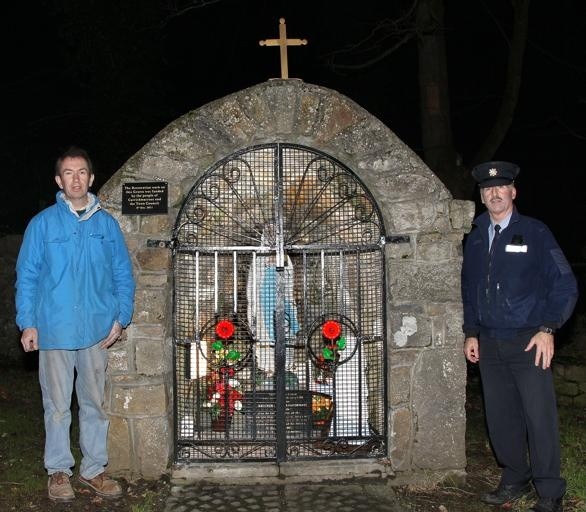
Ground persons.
[244,224,300,377]
[14,145,135,501]
[460,160,579,510]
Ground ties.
[490,224,502,251]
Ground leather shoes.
[77,469,124,497]
[47,471,77,504]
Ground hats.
[469,160,523,189]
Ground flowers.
[311,318,346,389]
[206,318,245,404]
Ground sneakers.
[480,476,533,506]
[525,495,563,512]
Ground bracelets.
[538,326,556,335]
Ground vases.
[207,400,235,432]
